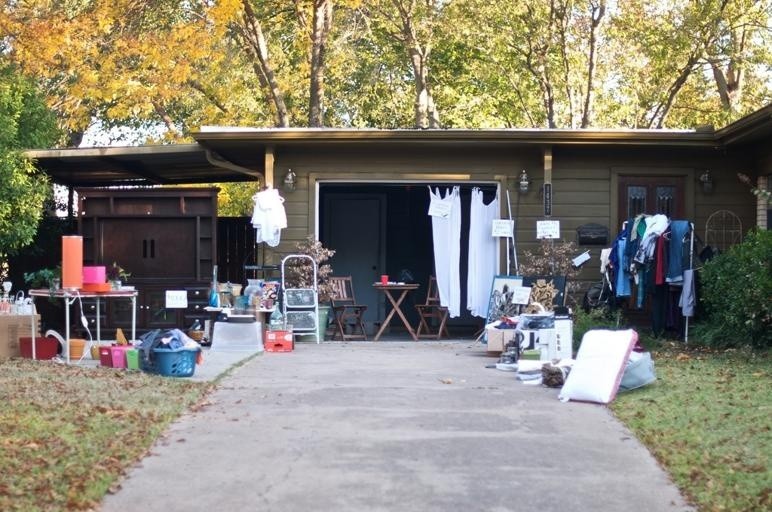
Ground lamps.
[697,165,716,196]
[282,167,300,196]
[60,234,83,291]
[513,168,534,197]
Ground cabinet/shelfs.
[72,186,222,342]
[215,213,258,289]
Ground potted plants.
[283,234,339,344]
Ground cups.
[380,276,388,285]
[49,277,59,292]
[115,280,122,291]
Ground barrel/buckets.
[69,338,86,361]
[125,349,139,369]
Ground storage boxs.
[123,347,148,371]
[1,313,45,363]
[98,345,134,369]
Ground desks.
[200,305,280,340]
[24,286,139,366]
[370,281,421,342]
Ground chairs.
[412,274,451,342]
[279,251,322,346]
[325,272,372,341]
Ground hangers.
[252,181,286,204]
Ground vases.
[108,279,122,289]
[244,277,263,297]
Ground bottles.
[0,292,33,315]
[187,319,203,343]
[270,302,283,331]
[501,347,517,364]
[210,265,280,317]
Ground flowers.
[102,261,133,283]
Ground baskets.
[135,341,201,378]
